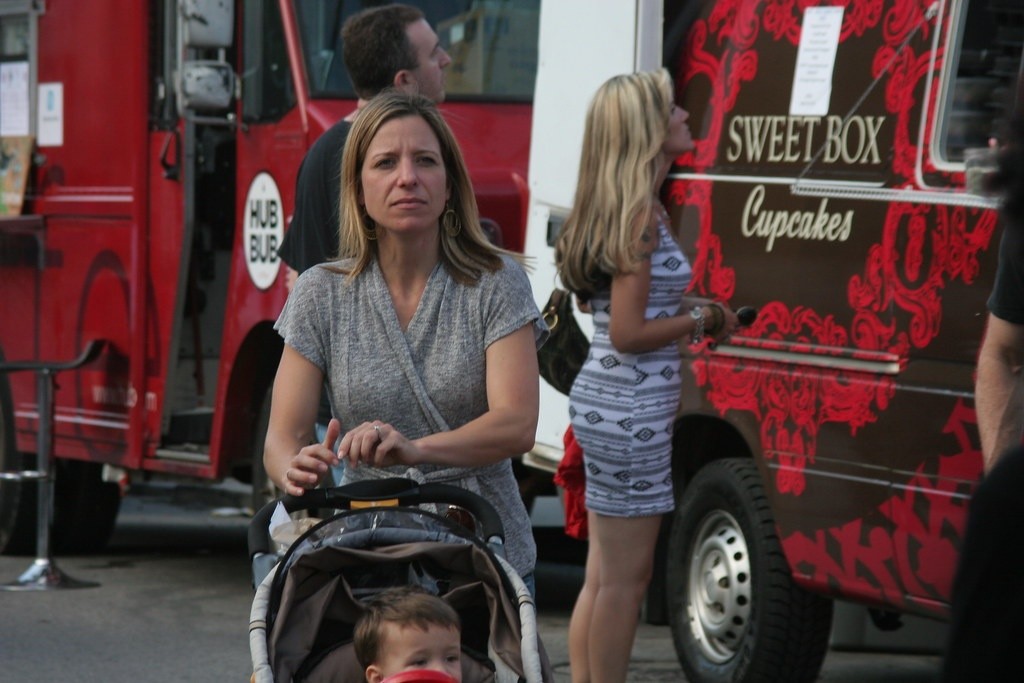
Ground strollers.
[245,477,555,683]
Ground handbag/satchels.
[537,265,592,396]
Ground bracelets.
[689,305,705,345]
[706,304,725,335]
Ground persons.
[266,2,453,298]
[352,587,466,679]
[943,2,1024,683]
[554,66,739,679]
[265,86,546,606]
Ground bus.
[510,0,1024,683]
[0,0,541,559]
